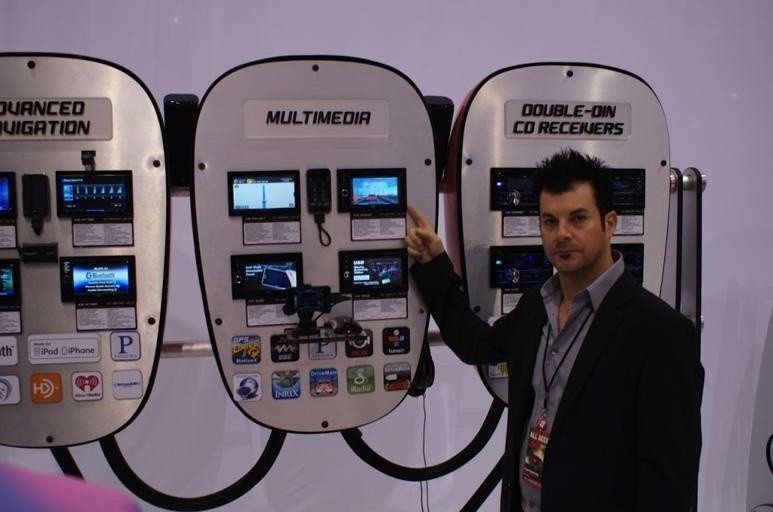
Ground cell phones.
[285,285,331,312]
[20,174,50,218]
[307,167,332,213]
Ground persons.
[406,146,705,511]
